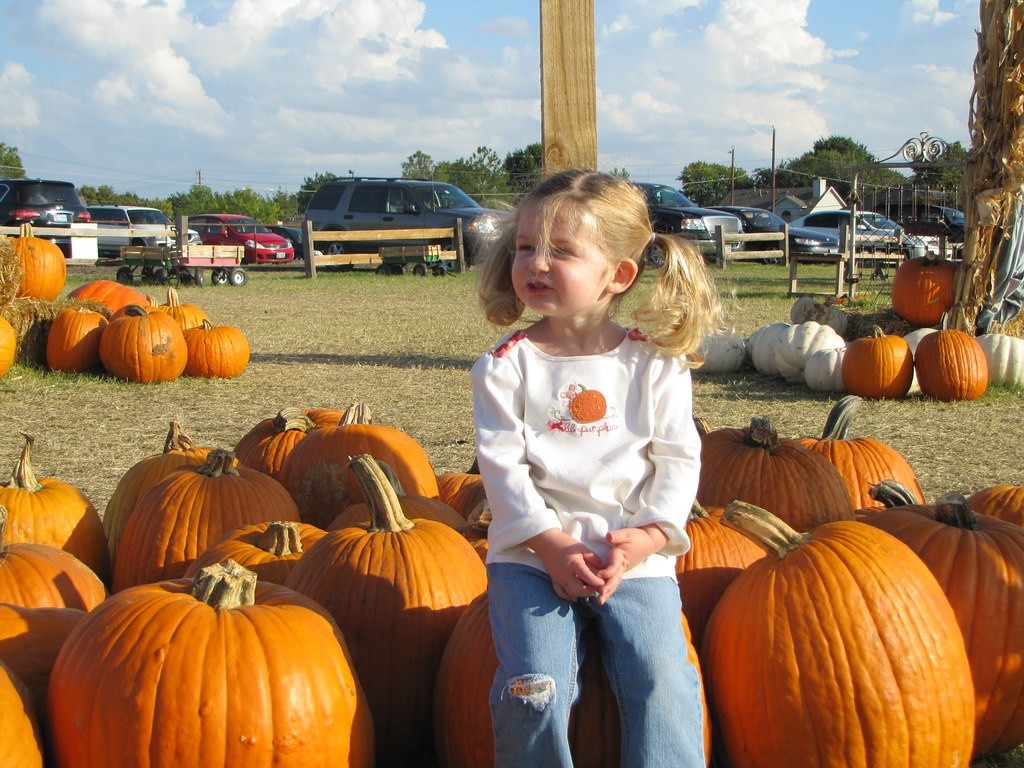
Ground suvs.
[86,204,203,257]
[300,176,514,272]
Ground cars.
[0,178,91,268]
[636,183,744,270]
[878,200,966,259]
[790,211,927,267]
[187,215,294,265]
[708,206,838,265]
[267,226,304,260]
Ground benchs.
[787,223,904,300]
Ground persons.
[470,156,743,768]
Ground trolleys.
[117,227,250,286]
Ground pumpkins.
[6,222,67,301]
[46,280,250,383]
[677,395,1024,768]
[688,251,1024,402]
[0,403,621,768]
[0,316,16,378]
